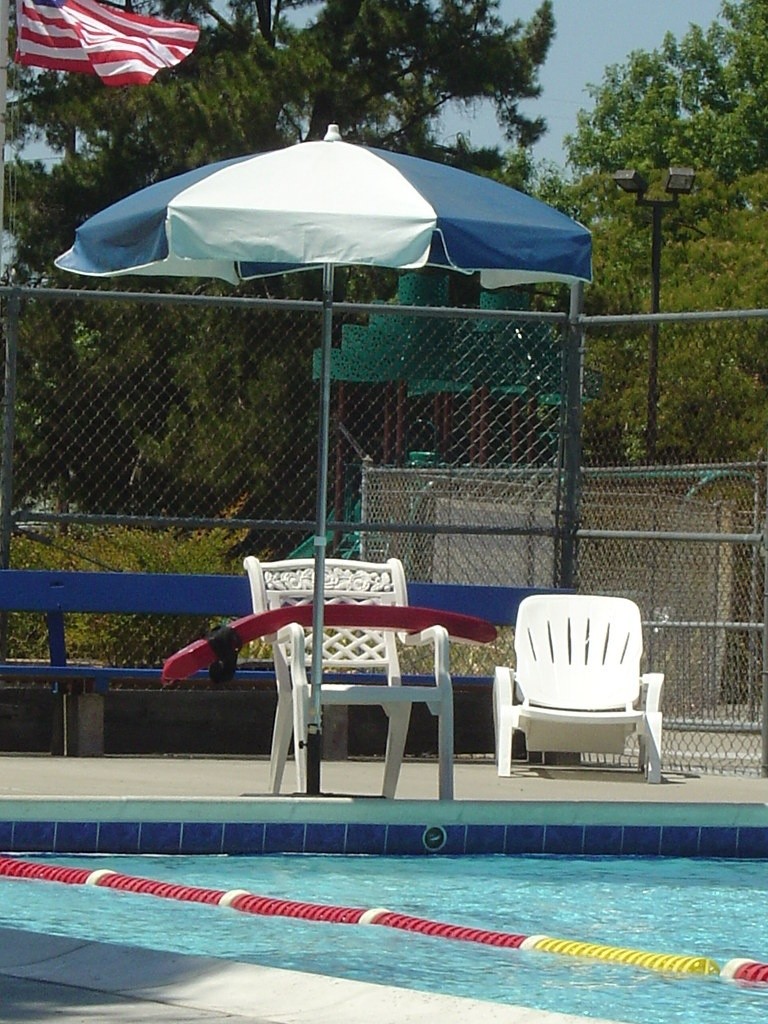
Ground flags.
[13,0,199,86]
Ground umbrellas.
[55,125,592,794]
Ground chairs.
[492,594,662,785]
[244,557,455,799]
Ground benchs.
[0,568,583,766]
